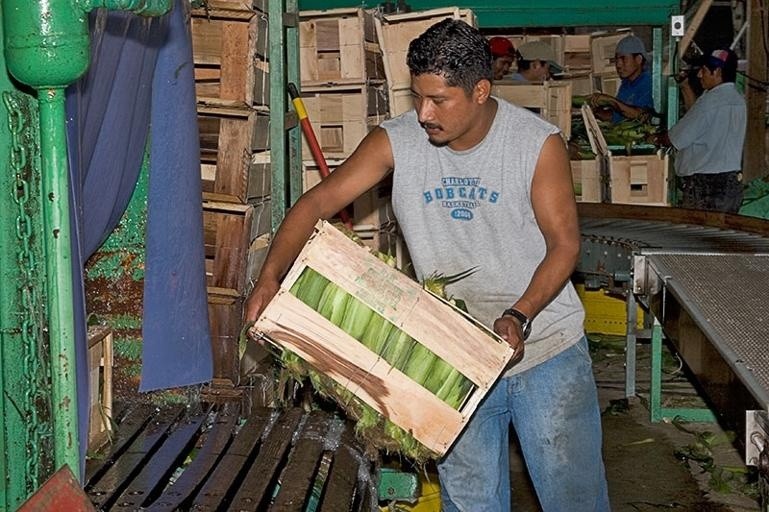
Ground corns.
[277,221,476,464]
[568,95,660,197]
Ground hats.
[704,46,737,68]
[489,37,515,57]
[517,41,565,73]
[616,36,650,63]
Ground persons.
[512,40,565,114]
[654,47,748,214]
[585,34,653,125]
[489,36,515,80]
[244,18,611,512]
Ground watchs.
[502,308,532,338]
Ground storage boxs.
[254,221,515,459]
[88,326,112,460]
[83,0,687,407]
